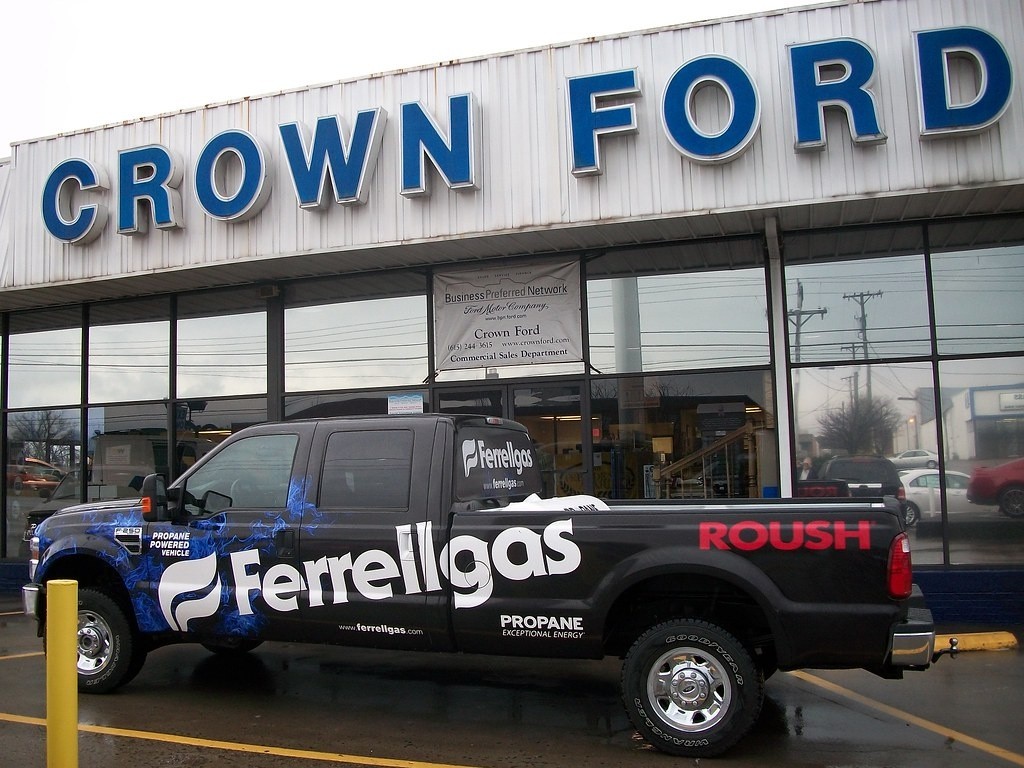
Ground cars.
[966,457,1024,518]
[5,455,72,500]
[887,450,940,469]
[897,470,1012,527]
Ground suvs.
[23,465,164,555]
[807,453,907,523]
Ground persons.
[796,456,816,480]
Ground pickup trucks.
[22,411,958,759]
